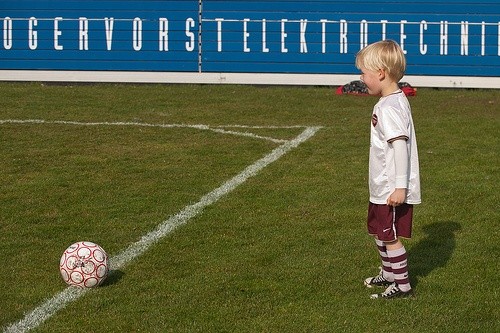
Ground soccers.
[58,242,110,290]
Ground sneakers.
[370,282,414,301]
[363,274,395,288]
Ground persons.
[356,41,422,300]
